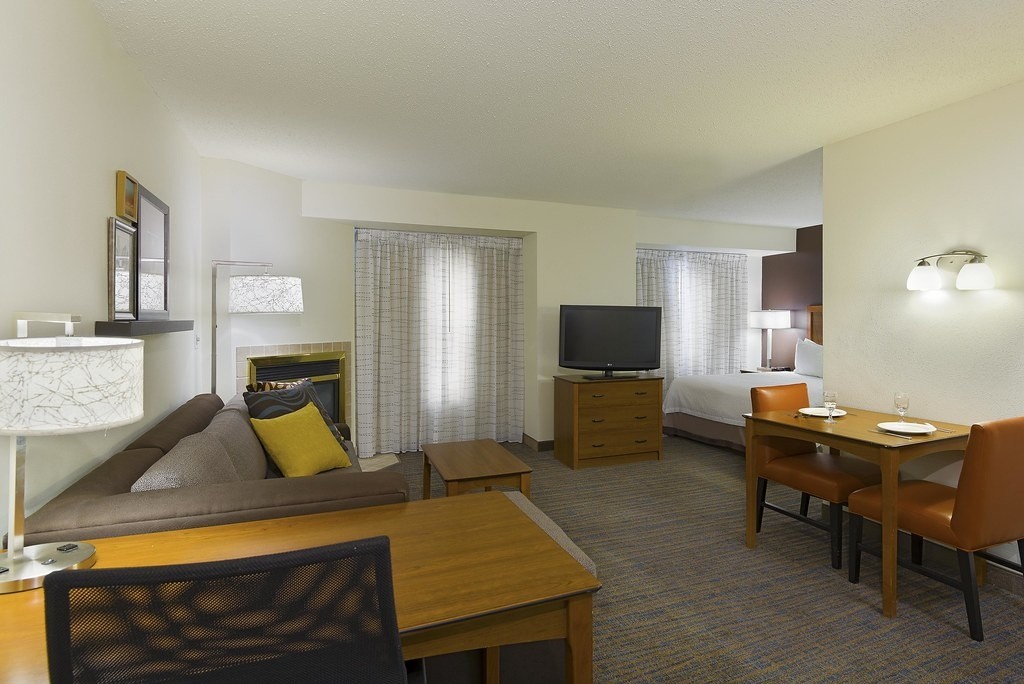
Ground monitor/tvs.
[559,305,662,380]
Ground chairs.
[42,537,403,683]
[750,384,902,568]
[847,417,1024,642]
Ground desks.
[740,404,972,615]
[0,491,604,684]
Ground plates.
[877,422,938,433]
[798,407,847,417]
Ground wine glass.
[822,391,838,423]
[893,392,910,428]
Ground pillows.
[250,402,353,478]
[246,377,345,442]
[242,379,349,480]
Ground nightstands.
[740,366,794,374]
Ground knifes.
[868,430,912,440]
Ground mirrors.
[133,184,170,319]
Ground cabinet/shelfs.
[552,372,663,471]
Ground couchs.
[3,394,409,550]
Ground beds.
[664,305,823,452]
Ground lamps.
[0,312,143,594]
[749,309,790,370]
[211,260,302,394]
[905,249,994,293]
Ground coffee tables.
[419,439,532,499]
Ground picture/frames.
[106,216,137,320]
[114,171,138,220]
